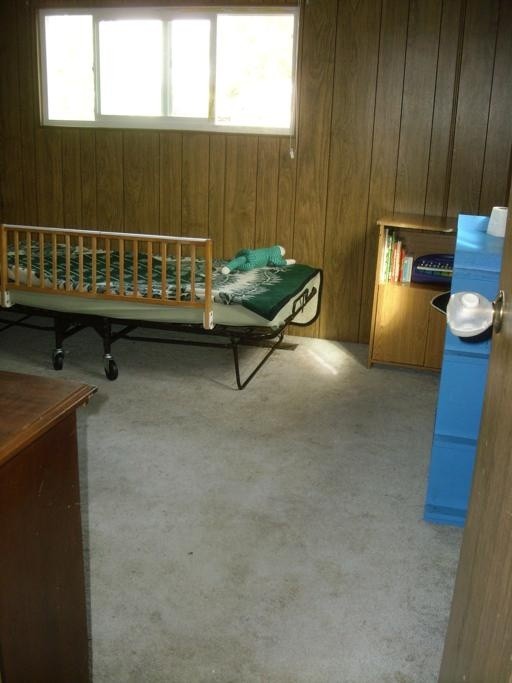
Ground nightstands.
[366,214,459,375]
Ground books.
[382,228,413,284]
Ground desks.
[0,370,99,683]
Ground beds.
[0,221,325,391]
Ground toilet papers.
[486,206,508,239]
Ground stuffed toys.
[222,244,299,275]
